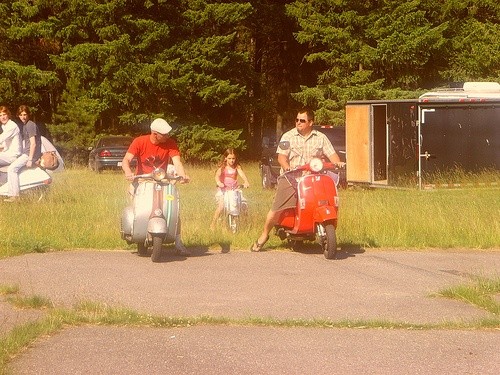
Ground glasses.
[295,119,305,123]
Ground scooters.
[0,145,60,196]
[121,156,187,264]
[273,143,347,258]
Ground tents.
[22,136,66,172]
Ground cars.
[85,135,140,172]
[258,125,347,191]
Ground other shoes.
[4,196,20,203]
[177,245,191,256]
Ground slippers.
[250,235,269,252]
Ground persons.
[4,105,41,202]
[208,147,249,232]
[0,106,23,167]
[122,118,191,256]
[249,108,344,252]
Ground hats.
[150,118,172,134]
